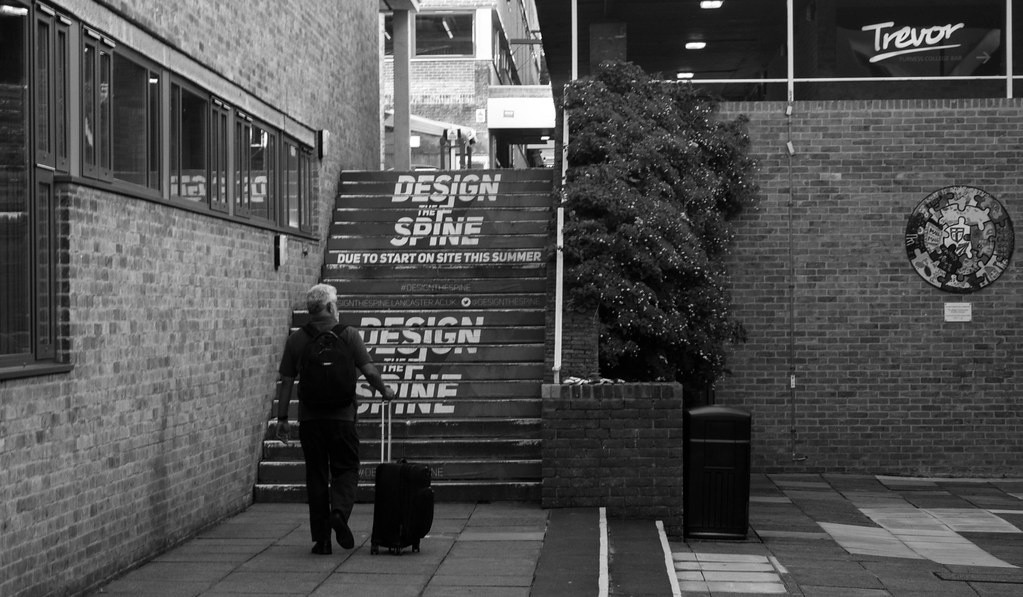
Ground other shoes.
[329,512,355,550]
[311,538,333,556]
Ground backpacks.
[297,319,359,421]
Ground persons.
[276,284,394,554]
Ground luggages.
[370,400,435,555]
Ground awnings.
[487,97,556,129]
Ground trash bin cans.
[683,406,752,541]
